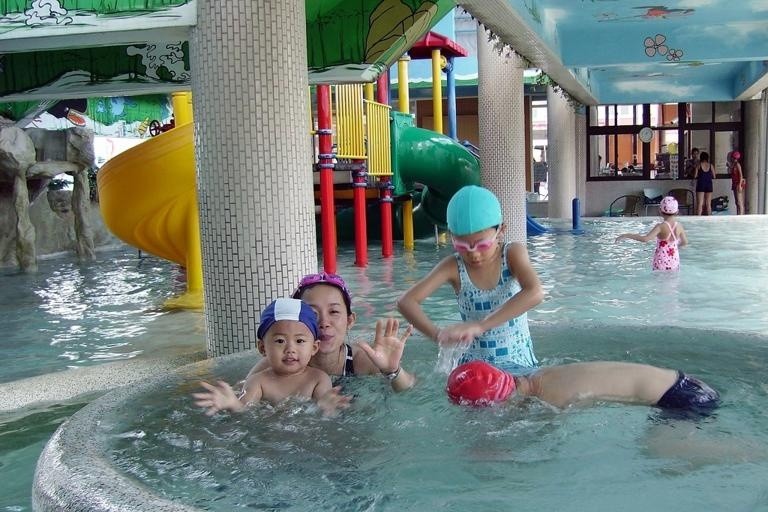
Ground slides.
[314,127,479,242]
[97,120,202,265]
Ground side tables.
[642,203,661,216]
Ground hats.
[731,150,741,159]
[288,272,353,308]
[659,196,679,214]
[445,361,519,410]
[255,296,321,340]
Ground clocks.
[639,127,654,143]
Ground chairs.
[609,195,641,217]
[668,189,695,215]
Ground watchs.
[386,366,402,381]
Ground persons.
[243,271,415,394]
[726,151,745,215]
[694,152,716,215]
[394,186,544,374]
[446,360,766,475]
[614,195,689,273]
[685,148,700,179]
[620,164,636,174]
[191,297,352,420]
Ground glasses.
[450,224,503,254]
[299,271,347,289]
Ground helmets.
[445,184,503,236]
